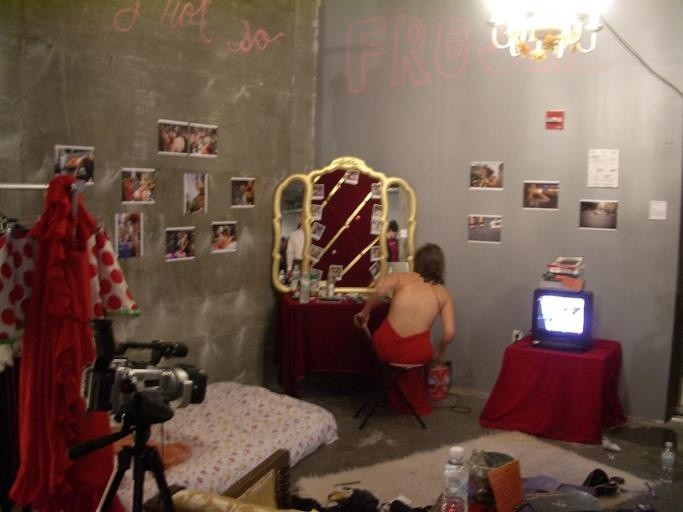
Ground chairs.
[352,325,428,430]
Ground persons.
[385,220,401,263]
[59,125,255,261]
[479,167,496,188]
[525,184,551,207]
[352,242,456,417]
[470,216,484,228]
[285,223,306,281]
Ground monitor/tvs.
[532,287,594,352]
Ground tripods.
[94,444,175,512]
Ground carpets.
[296,429,656,512]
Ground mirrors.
[270,156,417,296]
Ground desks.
[279,294,391,398]
[502,334,620,443]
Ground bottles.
[291,264,300,288]
[442,447,469,512]
[660,442,675,485]
[328,273,336,297]
[299,271,319,303]
